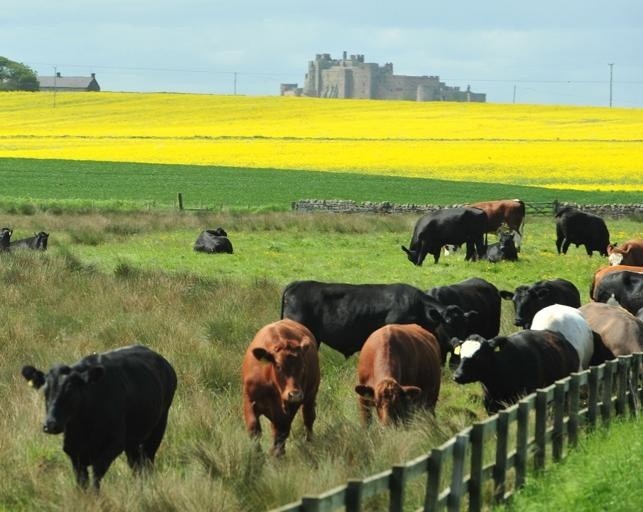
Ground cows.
[531,304,595,393]
[449,329,581,417]
[0,228,13,254]
[442,198,526,257]
[552,205,618,258]
[576,303,642,382]
[241,317,320,457]
[401,207,489,267]
[355,323,440,432]
[425,278,503,340]
[9,227,49,252]
[192,227,234,254]
[589,265,643,315]
[22,345,177,493]
[499,277,582,330]
[280,280,464,370]
[472,231,520,262]
[607,240,642,264]
